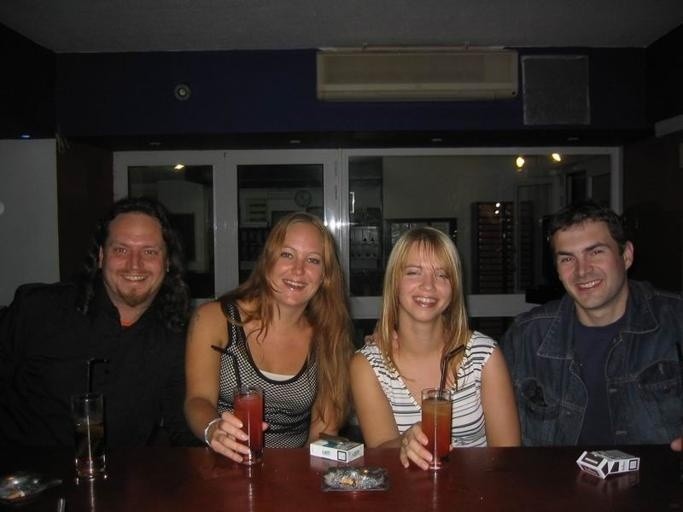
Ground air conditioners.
[315,48,517,100]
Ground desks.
[1,444,649,512]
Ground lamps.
[514,154,575,171]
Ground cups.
[421,387,453,471]
[232,385,264,467]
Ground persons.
[502,199,681,452]
[0,196,206,468]
[346,227,522,470]
[185,212,355,464]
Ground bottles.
[69,391,108,485]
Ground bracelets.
[204,418,220,444]
